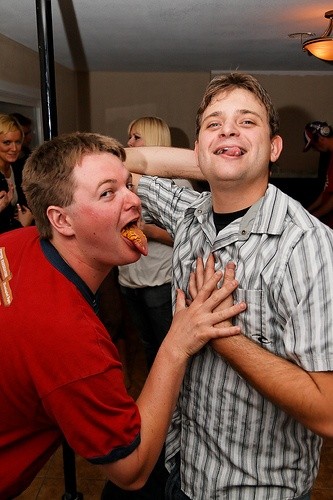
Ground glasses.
[308,143,315,148]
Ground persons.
[108,117,195,367]
[296,121,333,220]
[0,109,39,227]
[117,71,333,500]
[0,133,250,500]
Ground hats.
[302,121,326,153]
